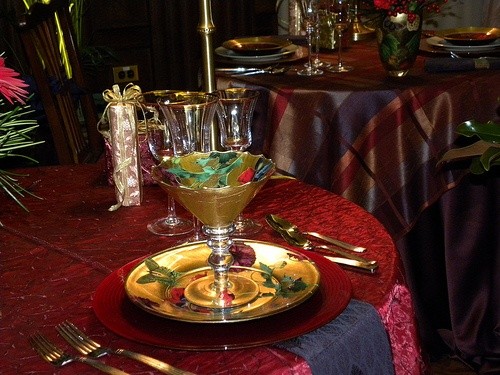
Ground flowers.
[356,0,449,29]
[0,50,43,214]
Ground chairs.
[17,0,107,165]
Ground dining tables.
[0,164,419,375]
[215,29,500,371]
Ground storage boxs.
[97,84,159,211]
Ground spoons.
[266,214,366,253]
[217,65,290,77]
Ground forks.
[27,332,131,375]
[280,230,377,273]
[54,320,196,375]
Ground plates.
[214,44,298,61]
[124,237,322,323]
[221,35,292,55]
[438,25,500,43]
[427,37,500,51]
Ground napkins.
[427,57,500,73]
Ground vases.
[376,10,424,77]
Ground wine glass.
[136,87,263,237]
[150,148,277,309]
[296,3,354,76]
